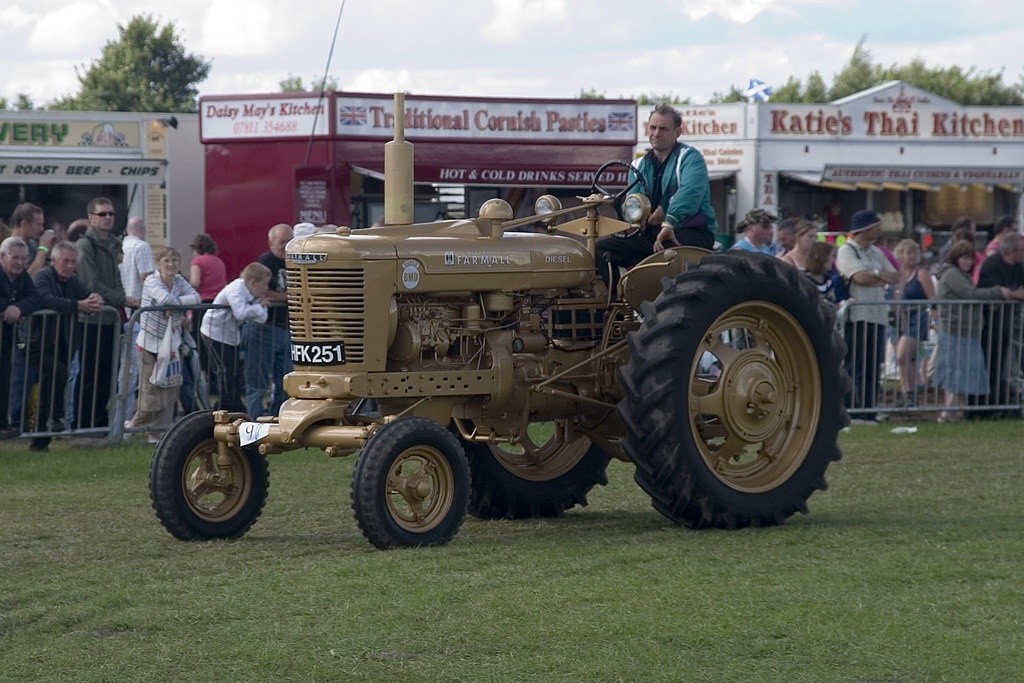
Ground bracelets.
[873,269,880,278]
[36,246,49,254]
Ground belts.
[266,320,289,329]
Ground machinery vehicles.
[146,91,854,551]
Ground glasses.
[5,253,30,262]
[90,211,115,217]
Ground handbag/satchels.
[826,243,860,301]
[149,316,185,389]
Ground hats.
[745,207,782,224]
[850,208,884,234]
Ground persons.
[595,106,719,304]
[0,194,1024,448]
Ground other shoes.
[0,424,22,440]
[123,420,134,441]
[896,390,917,407]
[147,433,159,443]
[875,412,890,423]
[27,441,49,453]
[47,418,66,433]
[938,410,966,425]
